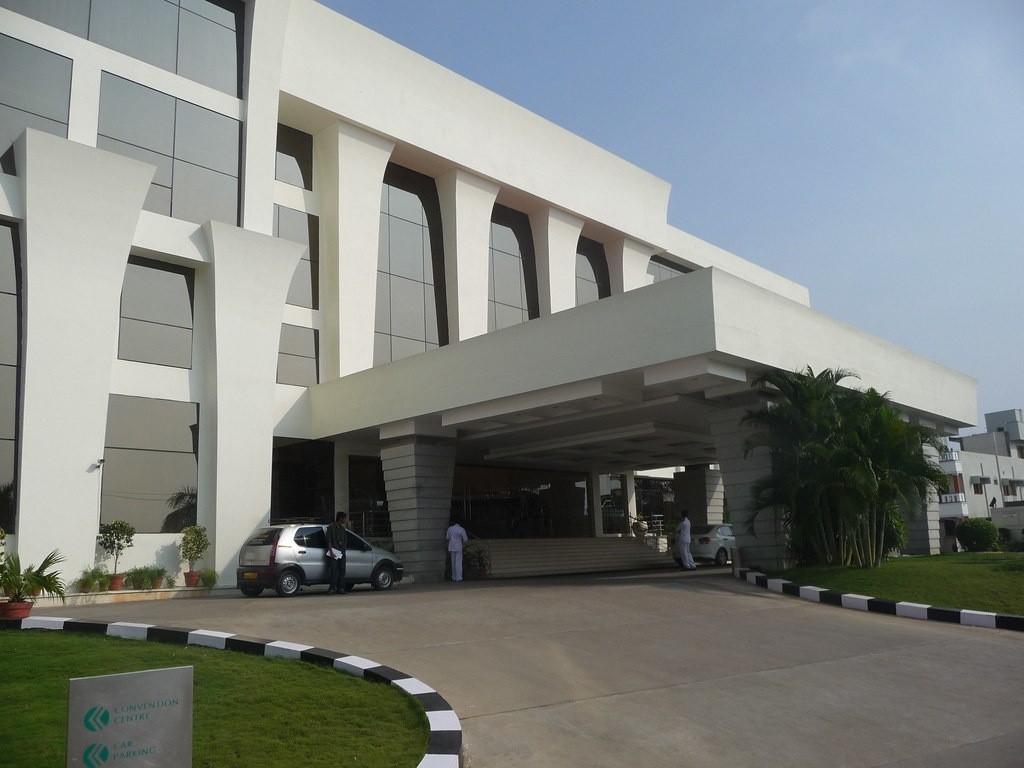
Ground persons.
[446,517,468,582]
[512,510,521,538]
[327,512,347,595]
[675,511,696,570]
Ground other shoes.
[337,589,346,594]
[681,567,696,571]
[327,590,336,595]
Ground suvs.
[236,517,404,598]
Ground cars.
[670,523,735,568]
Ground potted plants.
[461,543,490,581]
[632,514,648,537]
[179,525,211,588]
[0,546,67,620]
[76,521,166,591]
